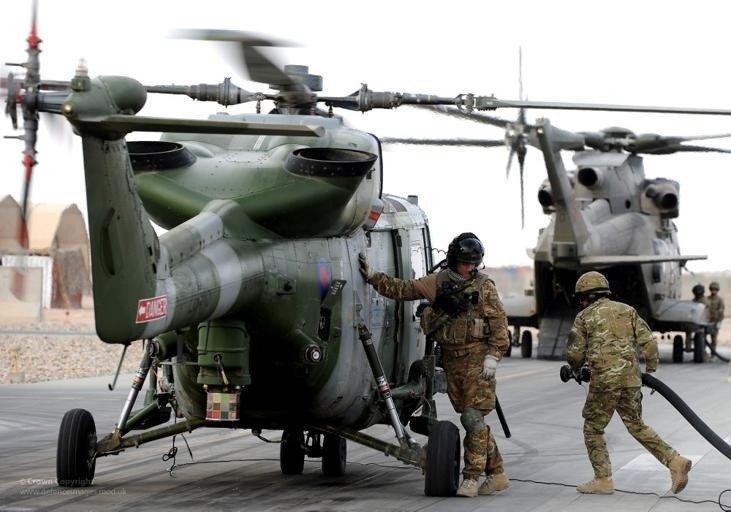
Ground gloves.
[358,252,377,280]
[481,355,498,379]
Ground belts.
[442,342,478,358]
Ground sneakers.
[577,476,616,495]
[457,477,479,497]
[478,472,510,494]
[668,455,693,493]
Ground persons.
[567,271,691,495]
[359,233,510,497]
[693,281,725,363]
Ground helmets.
[692,284,705,296]
[709,282,721,291]
[572,270,611,298]
[447,232,486,267]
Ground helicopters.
[377,44,731,365]
[1,2,463,495]
